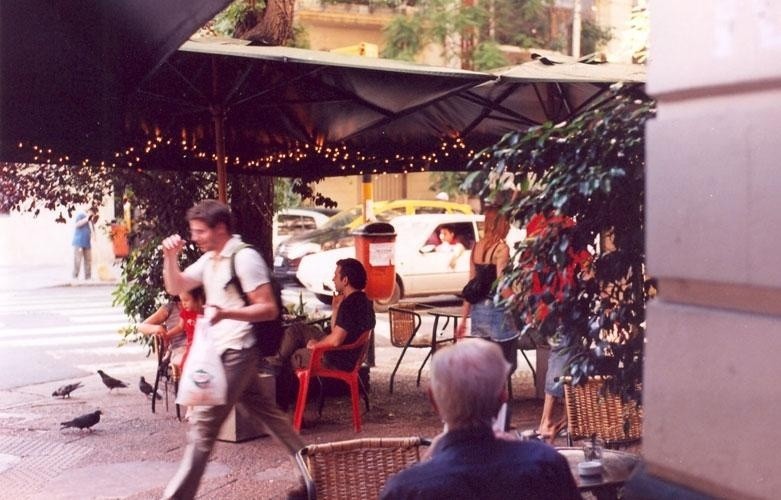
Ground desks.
[427,307,537,400]
[553,446,638,500]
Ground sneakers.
[254,355,283,376]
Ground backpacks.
[223,245,285,358]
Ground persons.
[157,200,318,500]
[419,224,468,267]
[521,204,609,442]
[161,287,206,373]
[458,207,536,431]
[68,203,98,286]
[141,294,188,363]
[378,333,586,500]
[258,255,377,430]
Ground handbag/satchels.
[463,276,493,304]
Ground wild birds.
[97,371,127,395]
[139,377,161,401]
[60,411,103,436]
[52,381,84,399]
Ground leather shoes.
[286,483,308,497]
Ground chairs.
[553,376,644,454]
[296,437,433,500]
[149,325,183,422]
[279,301,455,435]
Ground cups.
[583,439,603,464]
